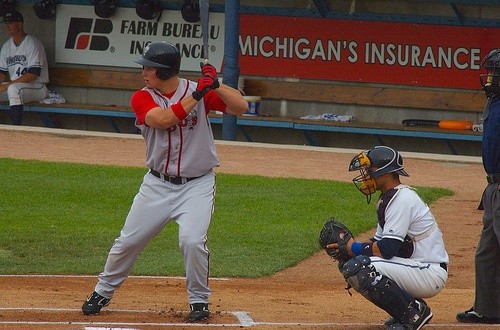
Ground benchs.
[0,68,486,155]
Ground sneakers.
[456,306,500,324]
[81,291,111,314]
[385,298,433,330]
[186,304,209,322]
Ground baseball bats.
[199,0,209,64]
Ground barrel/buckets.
[243,95,260,117]
[216,77,246,115]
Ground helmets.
[132,41,181,81]
[480,49,500,100]
[366,145,410,177]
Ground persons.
[327,145,449,330]
[455,49,500,326]
[82,41,248,324]
[0,10,50,126]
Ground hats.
[0,11,24,23]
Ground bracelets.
[361,243,373,256]
[351,241,362,256]
[170,99,187,121]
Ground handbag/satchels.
[477,183,489,210]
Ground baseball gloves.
[318,219,355,261]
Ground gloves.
[191,74,214,101]
[200,62,220,89]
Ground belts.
[486,175,500,185]
[439,263,448,271]
[150,169,203,184]
